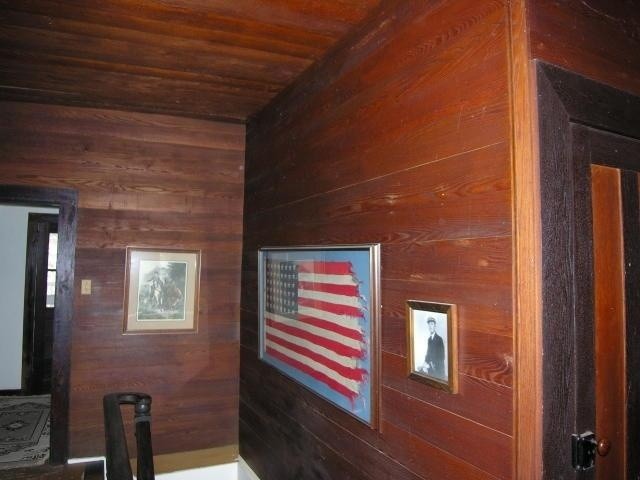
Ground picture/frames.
[257,244,383,432]
[121,245,203,337]
[403,299,458,395]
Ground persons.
[416,316,446,383]
[151,271,164,310]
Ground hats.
[427,317,437,323]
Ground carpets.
[0,393,51,472]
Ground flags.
[264,255,373,416]
